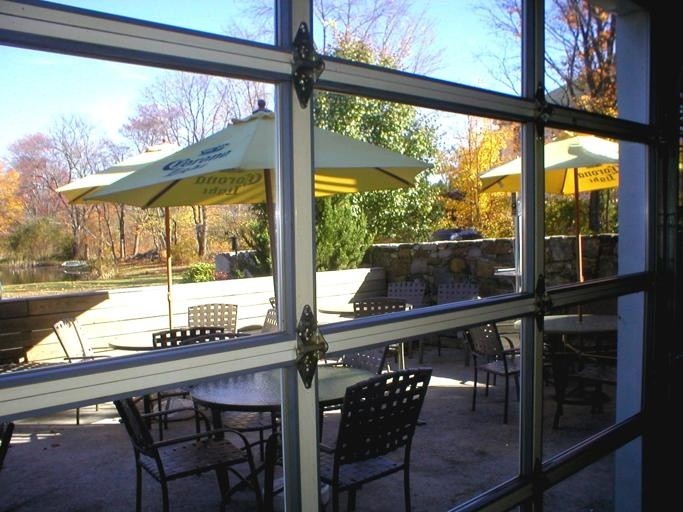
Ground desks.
[319,304,411,365]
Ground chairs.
[353,278,618,433]
[50,304,434,511]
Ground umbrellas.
[480,134,619,321]
[55,139,184,328]
[83,100,434,321]
